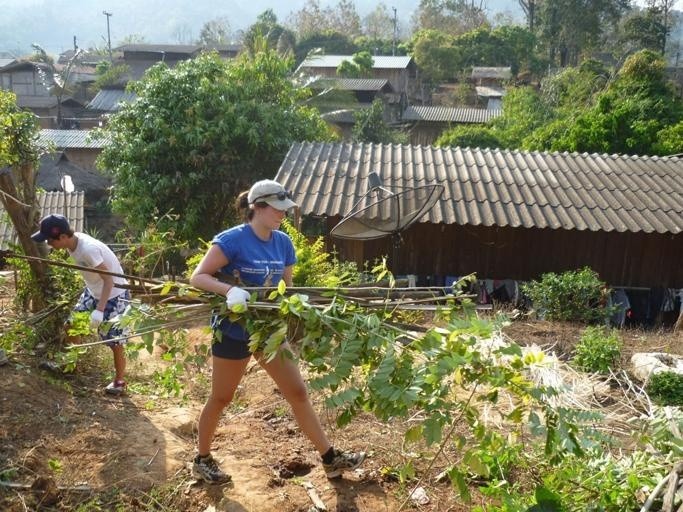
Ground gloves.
[225,286,250,313]
[87,308,103,330]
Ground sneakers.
[322,447,365,479]
[39,357,67,372]
[102,379,126,394]
[190,454,233,485]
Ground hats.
[29,213,70,243]
[246,178,298,212]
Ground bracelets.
[227,285,233,292]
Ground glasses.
[251,189,293,203]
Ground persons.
[30,212,133,397]
[188,179,371,487]
